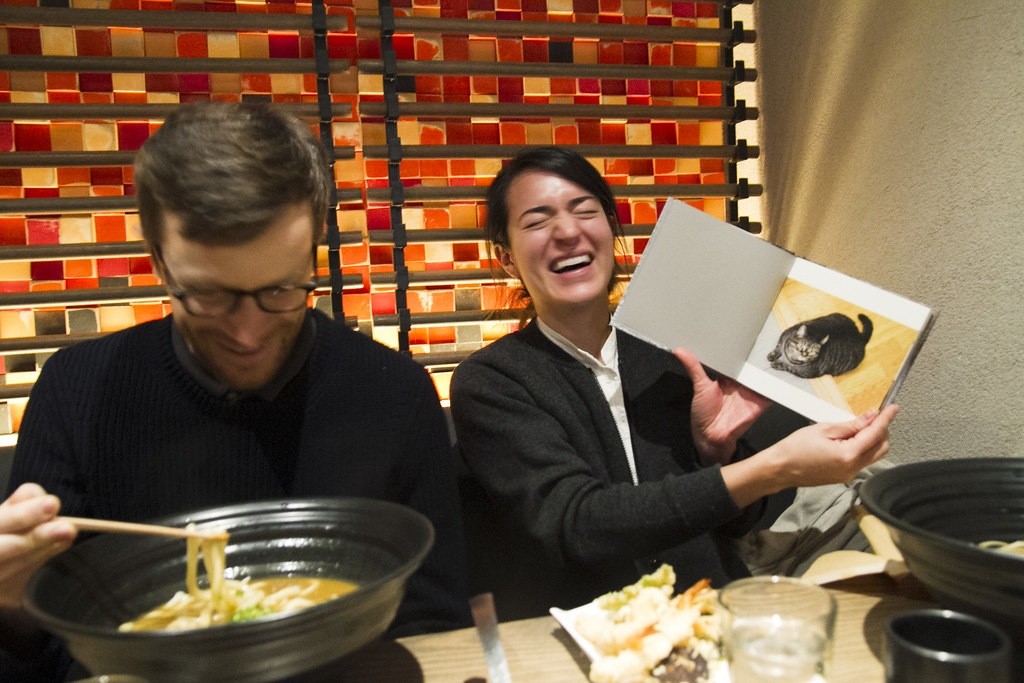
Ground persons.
[447,143,899,620]
[0,99,466,683]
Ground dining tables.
[397,570,1024,683]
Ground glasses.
[151,243,319,317]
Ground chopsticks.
[49,515,230,541]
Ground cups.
[884,608,1012,683]
[717,576,836,683]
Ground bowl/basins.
[860,458,1024,634]
[22,496,436,683]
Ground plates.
[549,584,830,683]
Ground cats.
[767,313,873,379]
